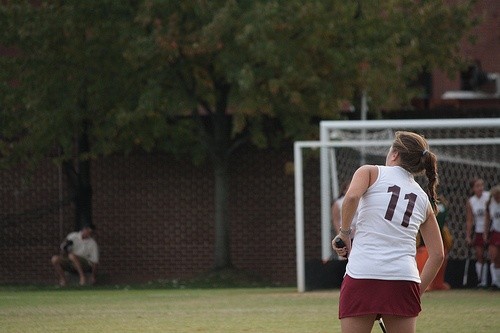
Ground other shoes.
[78,282,84,289]
[492,285,499,290]
[55,284,63,289]
[476,284,486,290]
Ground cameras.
[64,240,73,249]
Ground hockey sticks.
[462,224,475,286]
[334,238,391,333]
